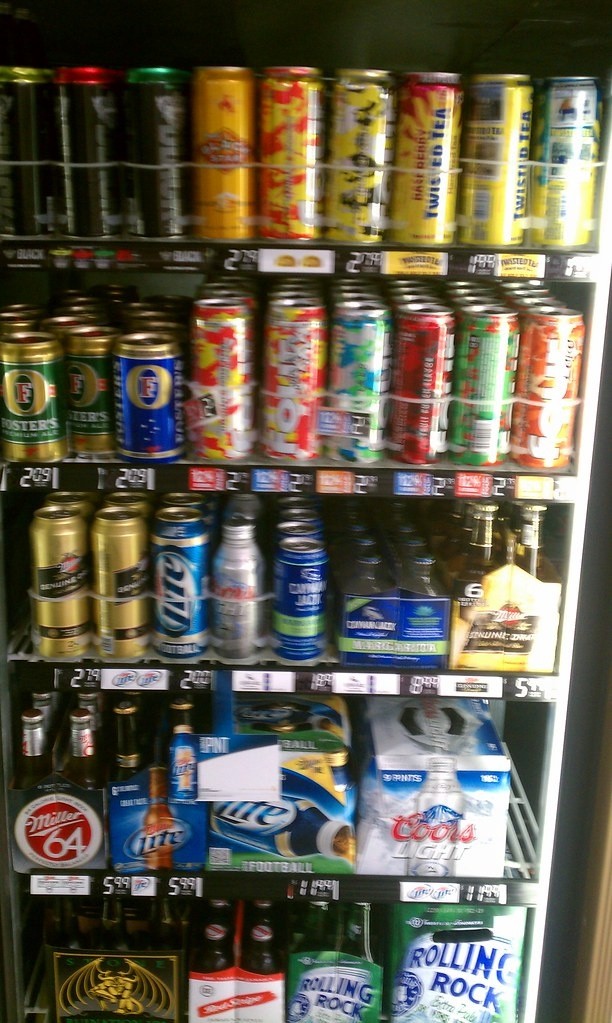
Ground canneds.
[1,68,602,663]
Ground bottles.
[7,681,205,884]
[22,890,384,1023]
[332,492,561,672]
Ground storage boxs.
[449,551,563,674]
[105,762,207,874]
[326,588,452,674]
[187,898,285,1023]
[206,693,355,875]
[283,902,382,1022]
[353,693,513,880]
[5,732,111,873]
[387,901,529,1023]
[39,942,188,1023]
